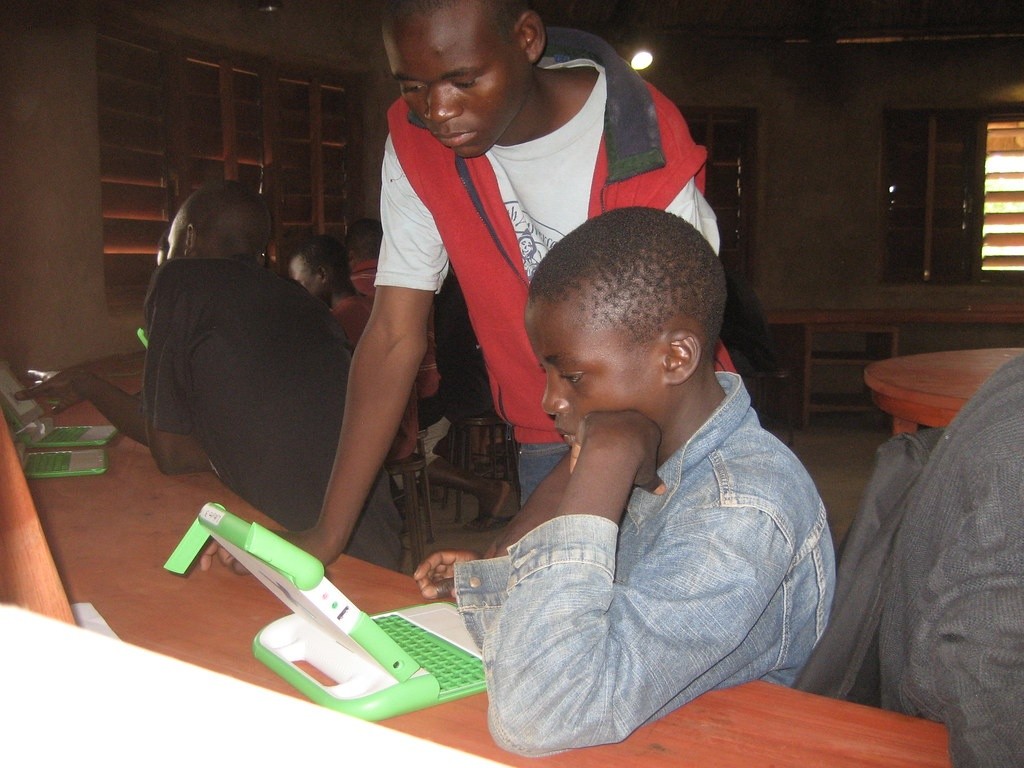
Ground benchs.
[759,306,1024,429]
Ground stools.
[739,366,793,446]
[387,452,433,573]
[441,412,520,525]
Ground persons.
[137,178,513,575]
[198,0,796,577]
[411,207,840,758]
[712,267,790,444]
[879,354,1024,768]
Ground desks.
[0,397,951,767]
[863,348,1024,434]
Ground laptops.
[163,501,493,723]
[0,360,119,479]
